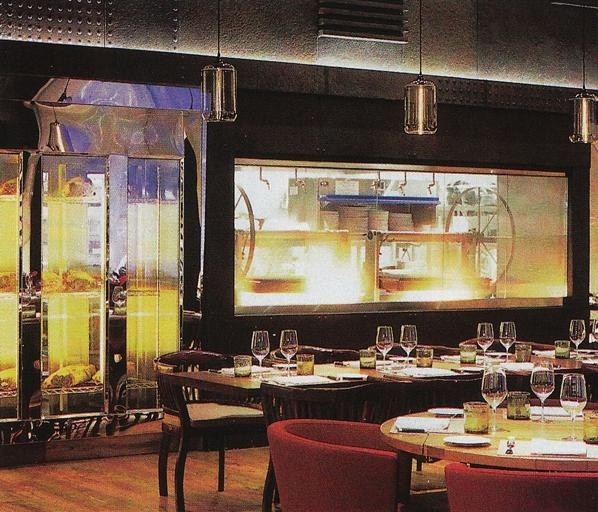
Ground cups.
[295,354,315,375]
[233,355,252,379]
[508,390,531,422]
[554,340,571,359]
[582,413,598,444]
[360,348,378,369]
[464,401,490,432]
[514,344,532,361]
[459,344,477,366]
[415,346,435,367]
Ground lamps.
[566,1,598,146]
[201,1,238,125]
[402,0,438,138]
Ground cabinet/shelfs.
[235,229,498,304]
[0,148,186,448]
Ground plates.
[319,203,415,234]
[428,408,465,415]
[444,435,490,446]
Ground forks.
[504,438,516,455]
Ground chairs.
[153,339,597,511]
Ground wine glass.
[477,322,496,366]
[279,329,299,379]
[528,367,555,425]
[399,324,419,368]
[499,322,516,364]
[482,367,508,424]
[251,331,270,379]
[569,319,586,360]
[376,326,395,371]
[560,373,588,442]
